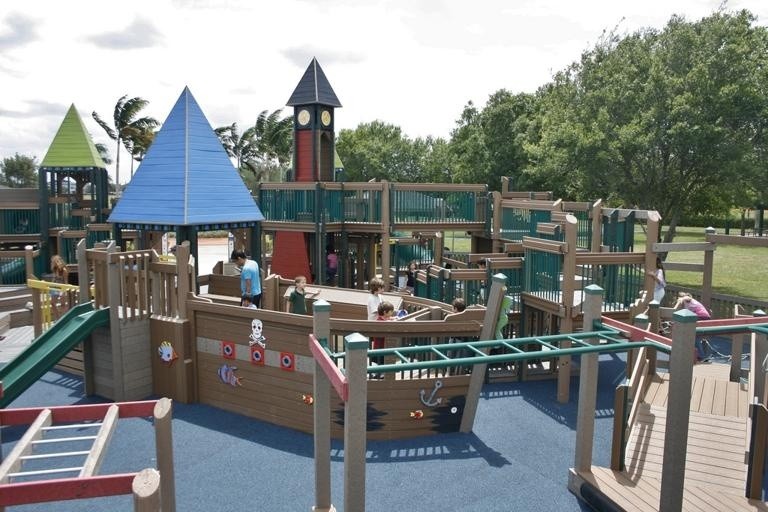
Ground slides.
[0,302,111,409]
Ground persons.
[324,246,338,286]
[241,291,257,310]
[365,277,399,352]
[50,255,69,285]
[371,300,395,381]
[672,290,713,364]
[286,274,322,315]
[230,248,261,307]
[444,297,469,376]
[647,257,668,333]
[476,257,488,306]
[405,260,426,295]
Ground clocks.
[298,109,310,126]
[321,110,332,127]
